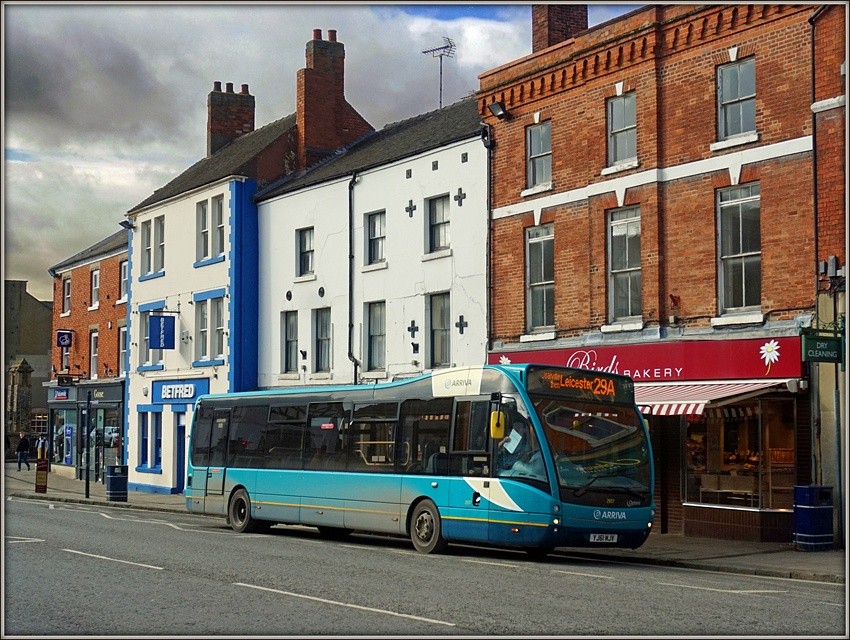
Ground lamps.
[118,221,137,232]
[487,101,513,121]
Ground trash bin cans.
[792,485,834,551]
[106,465,128,502]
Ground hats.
[39,432,45,436]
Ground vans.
[90,427,120,448]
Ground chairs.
[699,474,760,506]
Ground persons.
[34,434,49,458]
[501,420,529,471]
[15,431,31,471]
[57,415,64,463]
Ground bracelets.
[503,439,506,442]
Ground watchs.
[517,451,522,456]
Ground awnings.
[422,377,809,420]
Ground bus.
[185,363,656,555]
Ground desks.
[711,490,759,507]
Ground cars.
[58,424,87,446]
